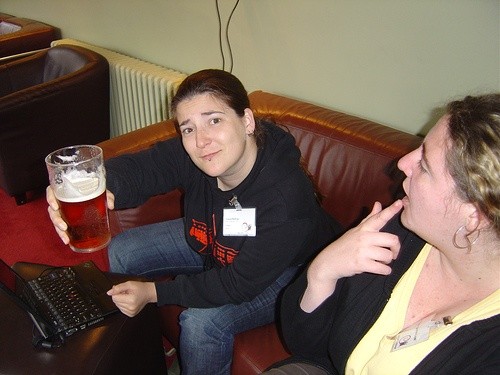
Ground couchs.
[76,89,426,374]
[1,12,62,52]
[2,42,111,205]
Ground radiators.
[50,38,188,137]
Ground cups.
[45,145,112,253]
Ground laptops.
[0,258,120,342]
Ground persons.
[45,68,341,375]
[259,92,500,375]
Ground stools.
[3,261,169,375]
